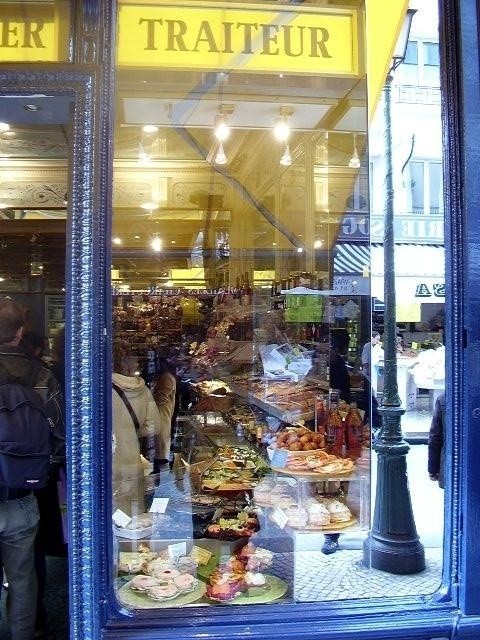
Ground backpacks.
[0,357,50,490]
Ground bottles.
[326,391,363,457]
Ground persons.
[0,299,66,640]
[143,357,177,474]
[298,330,382,555]
[112,342,163,524]
[428,391,445,489]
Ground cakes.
[254,478,290,504]
[129,542,256,601]
[277,478,352,527]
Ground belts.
[0,487,32,501]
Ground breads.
[276,427,325,451]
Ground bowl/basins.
[266,444,326,461]
[166,507,216,524]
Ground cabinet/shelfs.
[44,294,65,346]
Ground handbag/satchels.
[145,459,160,509]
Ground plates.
[118,550,289,609]
